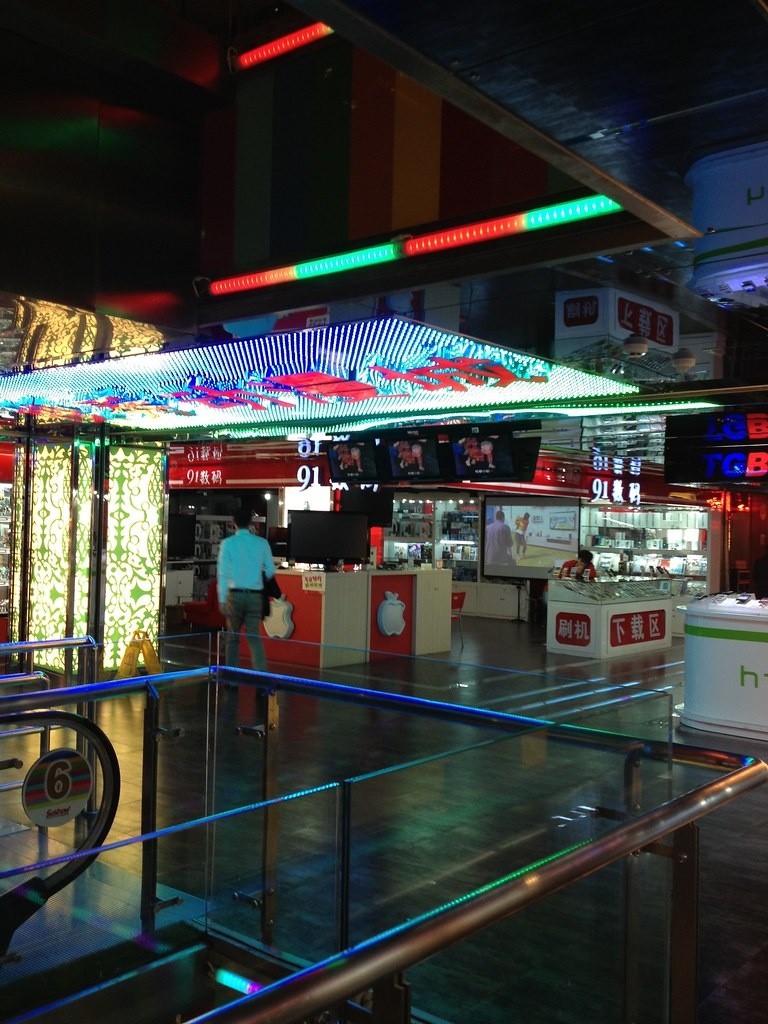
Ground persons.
[216,505,281,697]
[515,512,531,561]
[486,510,517,565]
[558,549,598,577]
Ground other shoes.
[224,685,238,691]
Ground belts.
[231,589,262,593]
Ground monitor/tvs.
[287,510,371,574]
[326,436,542,484]
[166,512,197,562]
[340,487,394,528]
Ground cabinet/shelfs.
[166,500,768,740]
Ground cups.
[402,563,407,570]
[289,558,295,571]
[436,559,442,569]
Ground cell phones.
[693,594,705,598]
[554,565,670,579]
[736,595,751,600]
[712,594,727,600]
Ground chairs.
[451,592,466,648]
[184,578,226,644]
